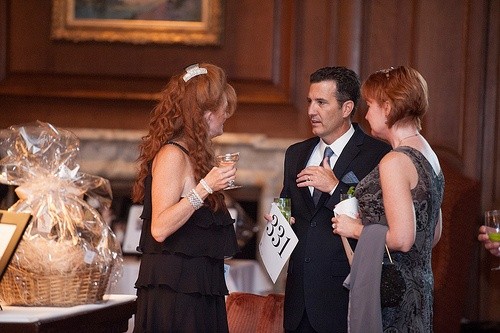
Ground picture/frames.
[49,0,226,47]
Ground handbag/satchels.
[379,243,406,309]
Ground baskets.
[1,194,114,306]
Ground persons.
[264,67,444,333]
[478,226,500,256]
[133,63,237,333]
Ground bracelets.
[186,188,204,210]
[200,178,213,194]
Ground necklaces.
[398,133,420,146]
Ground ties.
[313,147,334,209]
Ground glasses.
[384,66,393,81]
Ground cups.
[340,194,355,202]
[274,198,292,225]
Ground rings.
[332,224,336,228]
[307,175,310,181]
[229,180,235,186]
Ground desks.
[0,293,137,333]
[107,255,275,333]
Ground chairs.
[227,292,284,333]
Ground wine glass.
[485,210,500,270]
[217,152,243,190]
